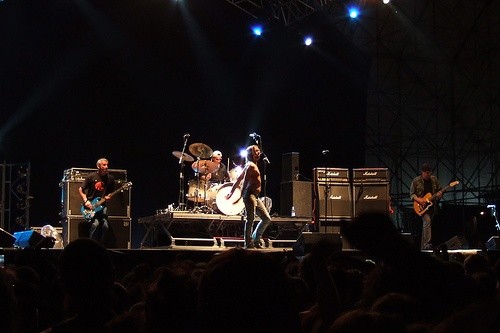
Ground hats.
[212,150,222,157]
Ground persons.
[410,159,445,249]
[299,209,500,333]
[79,158,129,246]
[0,220,311,333]
[209,150,230,183]
[224,145,272,249]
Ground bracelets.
[83,199,88,203]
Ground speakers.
[0,229,16,247]
[353,183,390,218]
[294,232,343,253]
[61,179,131,219]
[280,181,313,218]
[317,182,352,218]
[281,152,300,181]
[13,230,45,248]
[60,217,131,250]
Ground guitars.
[79,180,133,223]
[413,179,460,217]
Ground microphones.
[183,134,191,140]
[249,132,260,138]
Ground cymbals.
[188,143,213,158]
[171,151,195,162]
[191,160,219,175]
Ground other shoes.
[253,240,262,248]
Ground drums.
[209,183,245,216]
[226,164,244,183]
[186,178,214,202]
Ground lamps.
[16,165,29,227]
